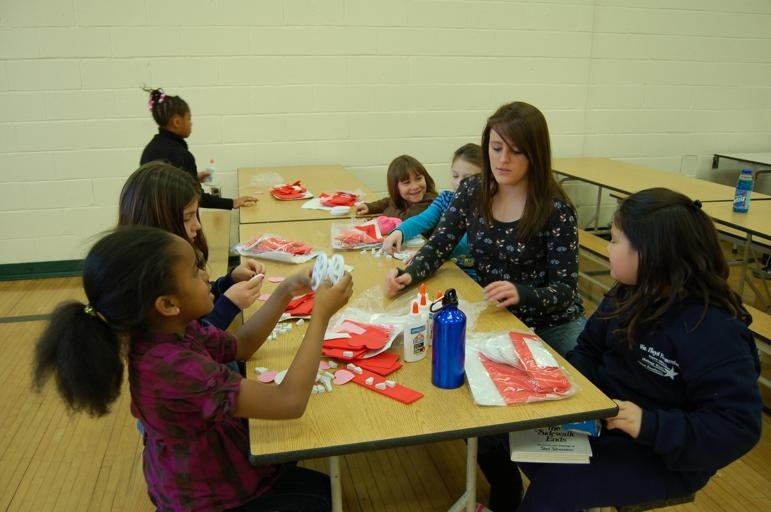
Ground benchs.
[197,206,231,281]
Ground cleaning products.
[404,283,444,363]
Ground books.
[509,425,592,464]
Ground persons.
[31,222,353,510]
[464,188,760,512]
[386,102,588,356]
[140,81,259,211]
[119,163,266,435]
[358,142,484,283]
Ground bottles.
[403,284,466,390]
[732,169,753,214]
[204,160,216,186]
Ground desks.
[240,217,620,511]
[237,165,374,221]
[553,155,771,512]
